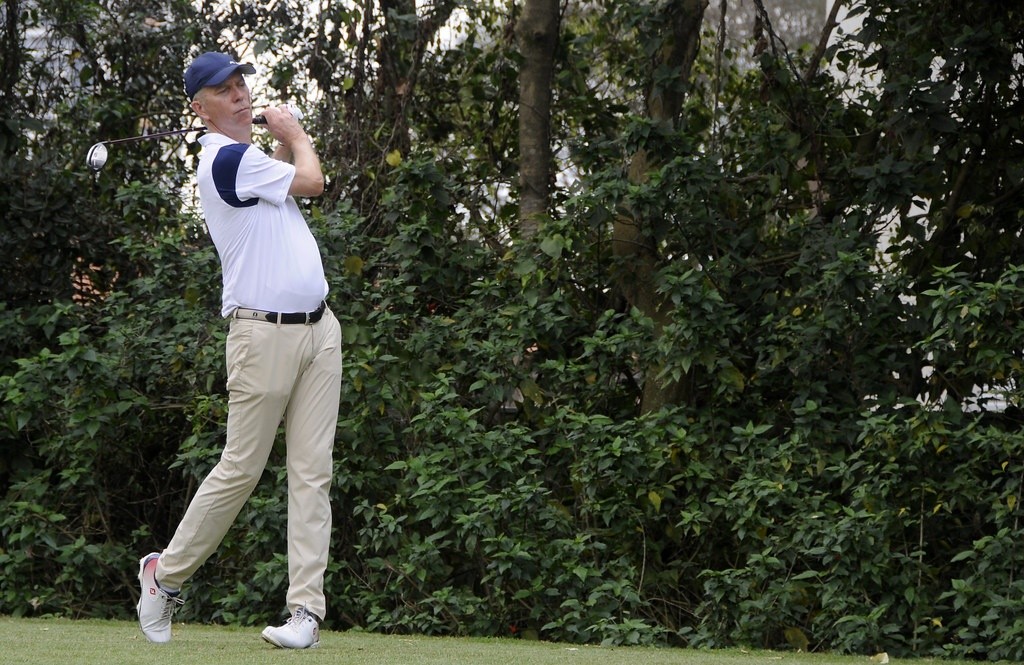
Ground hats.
[185,51,256,97]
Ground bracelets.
[278,143,284,146]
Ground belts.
[231,300,326,324]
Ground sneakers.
[262,600,319,649]
[135,552,185,643]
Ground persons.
[139,54,341,649]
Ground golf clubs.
[86,117,269,174]
